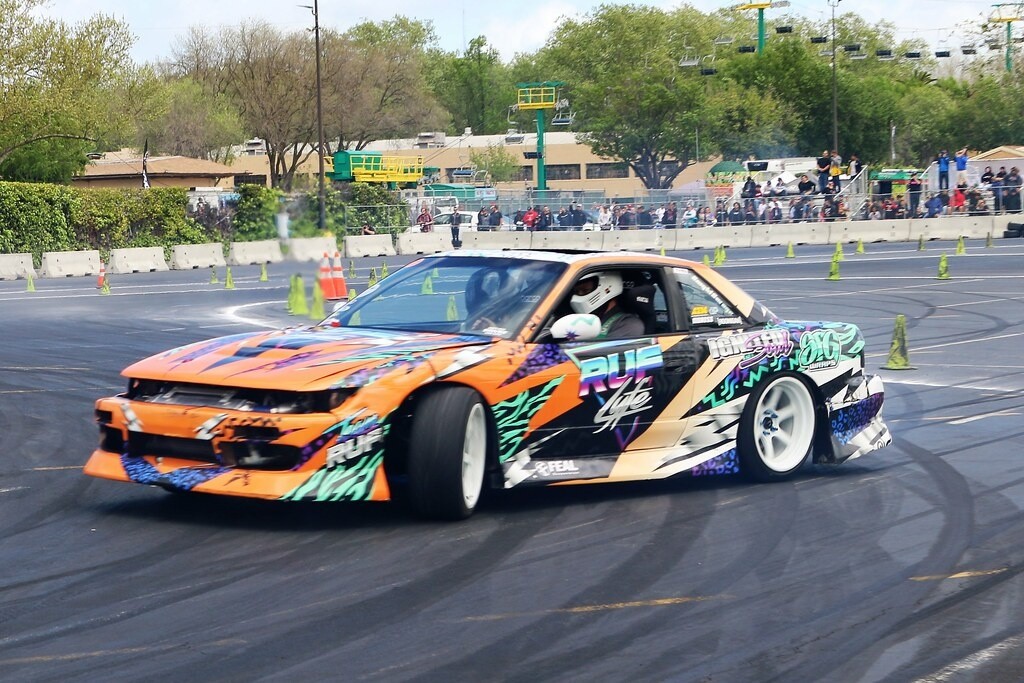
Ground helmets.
[570,271,623,318]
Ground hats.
[911,172,917,177]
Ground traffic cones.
[367,267,384,301]
[856,238,865,254]
[825,253,842,281]
[916,234,929,252]
[660,247,667,257]
[332,252,349,299]
[285,272,328,321]
[879,313,919,371]
[349,260,357,279]
[96,260,113,296]
[833,240,846,261]
[955,235,967,255]
[432,266,440,278]
[703,244,727,267]
[26,273,37,293]
[319,252,340,300]
[783,242,796,258]
[418,272,439,296]
[985,231,995,248]
[224,266,235,289]
[446,296,461,322]
[259,262,269,282]
[381,261,389,278]
[211,266,220,284]
[347,288,361,325]
[936,251,952,281]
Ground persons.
[197,198,204,210]
[741,176,787,197]
[937,150,949,190]
[472,271,645,343]
[477,204,503,231]
[362,222,375,235]
[860,195,922,220]
[450,207,462,241]
[846,153,861,195]
[799,175,815,195]
[682,194,847,228]
[513,201,587,231]
[906,171,922,211]
[416,208,434,233]
[822,181,836,202]
[953,147,968,188]
[818,149,842,193]
[422,201,429,211]
[925,166,1022,218]
[591,201,677,231]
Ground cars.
[83,248,893,520]
